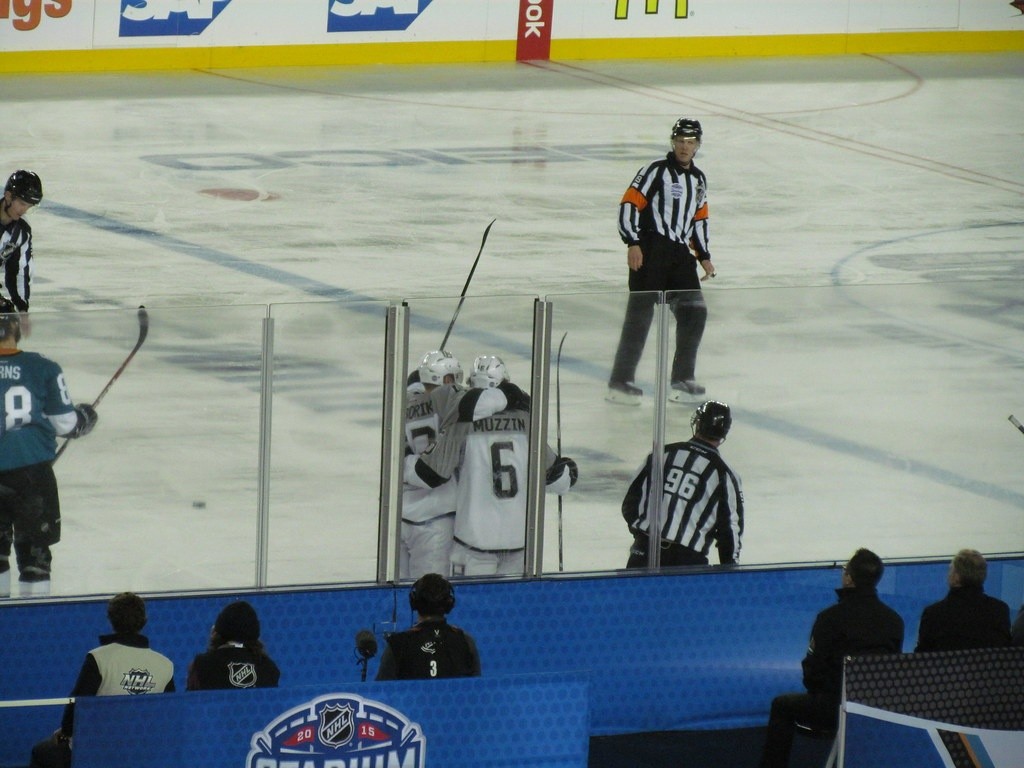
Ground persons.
[622,399,744,567]
[0,170,43,336]
[0,304,99,598]
[374,572,481,679]
[186,601,280,690]
[404,351,578,576]
[604,116,715,406]
[67,592,176,697]
[764,546,904,768]
[913,549,1012,653]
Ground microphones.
[356,630,377,658]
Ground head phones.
[409,580,456,613]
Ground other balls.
[193,501,206,508]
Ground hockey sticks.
[54,304,150,463]
[440,217,497,352]
[1006,413,1024,434]
[555,330,570,572]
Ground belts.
[638,535,687,549]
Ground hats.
[215,602,259,641]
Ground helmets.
[692,401,731,437]
[468,356,509,388]
[7,170,42,204]
[418,351,463,386]
[672,118,702,140]
[0,298,20,340]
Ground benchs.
[794,648,1024,768]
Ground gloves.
[498,381,529,409]
[561,456,578,485]
[72,404,98,439]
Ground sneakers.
[606,379,643,404]
[669,380,706,401]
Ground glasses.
[842,563,847,570]
